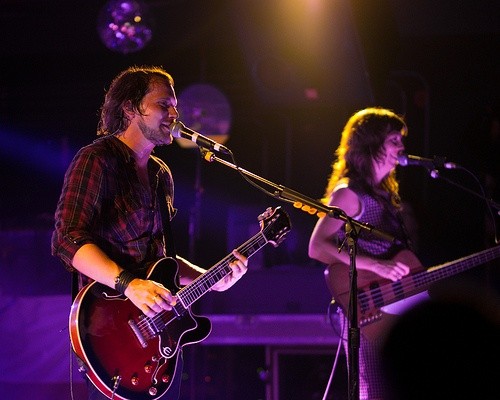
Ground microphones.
[171,121,230,154]
[399,153,458,170]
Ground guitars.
[66,205,293,400]
[324,242,500,347]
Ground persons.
[51,67,250,400]
[310,106,425,400]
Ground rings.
[152,294,160,300]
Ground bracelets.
[114,270,135,293]
